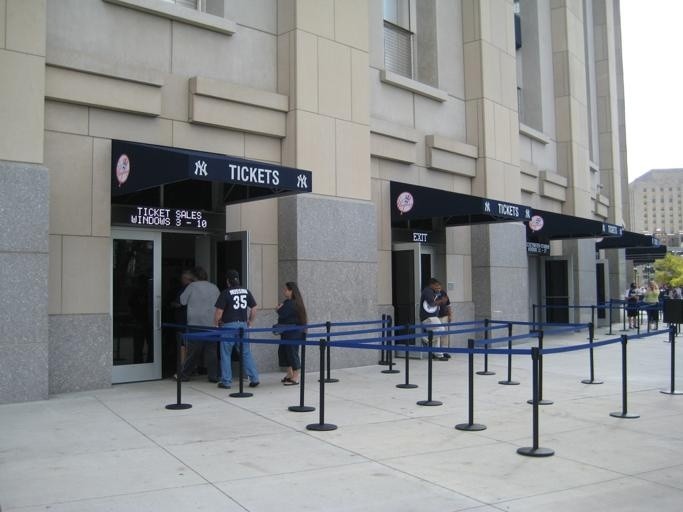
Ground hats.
[224,270,239,280]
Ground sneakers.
[249,381,258,387]
[218,382,232,389]
[432,353,450,361]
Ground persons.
[174,271,192,381]
[434,283,452,358]
[213,269,260,389]
[419,278,448,361]
[274,282,307,386]
[625,280,683,330]
[176,266,222,383]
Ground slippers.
[284,380,299,386]
[281,376,291,382]
